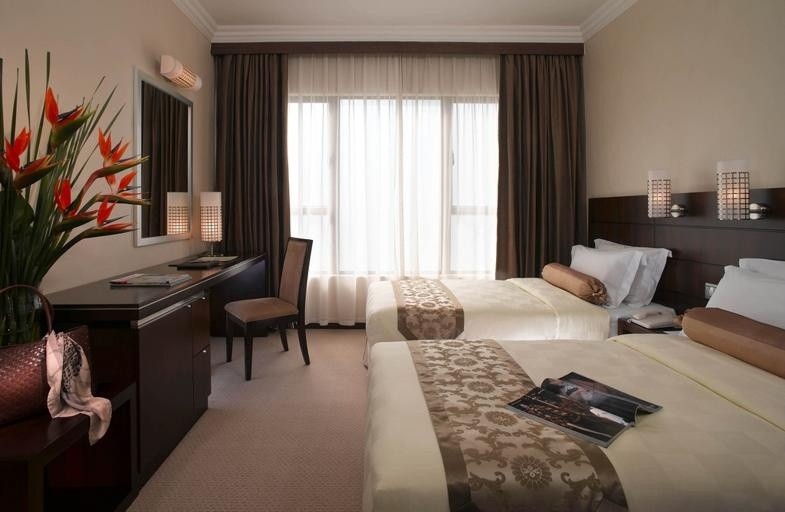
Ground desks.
[0,385,138,512]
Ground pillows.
[540,237,671,307]
[682,257,785,380]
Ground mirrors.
[132,68,194,246]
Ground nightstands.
[618,317,681,333]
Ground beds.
[360,257,785,512]
[363,234,673,365]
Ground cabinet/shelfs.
[40,250,273,484]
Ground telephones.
[631,308,676,329]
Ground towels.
[42,333,112,447]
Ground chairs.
[224,237,313,381]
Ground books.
[107,273,193,287]
[502,371,665,448]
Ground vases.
[0,323,90,426]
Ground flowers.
[0,47,151,344]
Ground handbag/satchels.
[0,284,93,424]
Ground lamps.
[160,54,202,93]
[192,191,239,262]
[717,160,765,221]
[646,170,685,219]
[167,191,188,234]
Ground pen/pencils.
[168,264,179,267]
